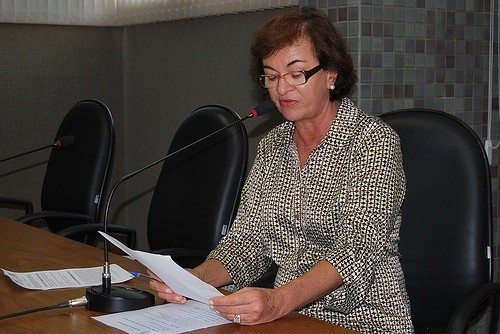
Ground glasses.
[259,64,325,88]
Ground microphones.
[86,99,275,313]
[0,136,75,163]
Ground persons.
[146,7,414,334]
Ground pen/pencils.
[131,272,163,284]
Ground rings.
[233,314,240,323]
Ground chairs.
[55,104,249,267]
[371,109,499,333]
[0,99,116,246]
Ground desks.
[0,216,363,334]
